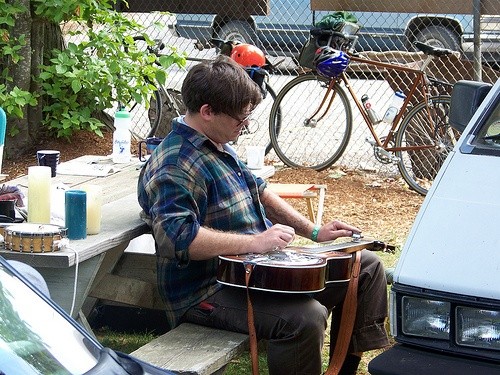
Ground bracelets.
[311,226,321,242]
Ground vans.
[366,74,500,374]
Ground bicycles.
[106,37,281,155]
[269,28,463,196]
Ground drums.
[0,222,69,252]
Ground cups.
[139,137,164,162]
[82,183,102,235]
[36,150,60,178]
[28,165,51,224]
[245,146,266,169]
[65,189,87,240]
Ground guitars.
[217,234,396,293]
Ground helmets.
[230,43,265,68]
[312,45,351,77]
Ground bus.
[175,0,500,62]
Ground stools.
[261,183,327,227]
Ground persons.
[137,54,391,375]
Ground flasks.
[361,93,382,124]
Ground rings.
[272,247,278,252]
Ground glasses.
[225,111,252,126]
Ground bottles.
[382,90,407,124]
[112,105,132,164]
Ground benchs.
[128,323,251,374]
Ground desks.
[0,154,152,320]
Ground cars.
[0,255,178,375]
